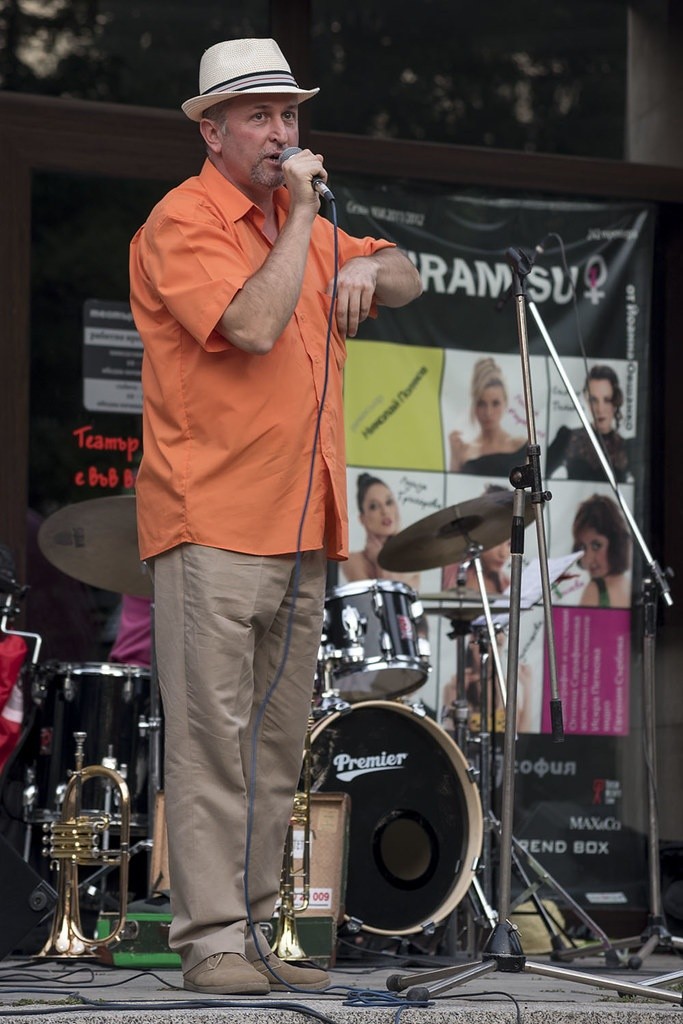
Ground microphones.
[279,147,335,204]
[496,235,551,311]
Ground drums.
[18,663,162,833]
[317,580,432,699]
[293,700,485,932]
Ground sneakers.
[252,952,331,992]
[183,952,270,994]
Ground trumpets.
[273,773,317,964]
[35,724,131,964]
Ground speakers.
[0,835,60,961]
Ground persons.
[338,471,423,596]
[435,623,539,735]
[107,593,152,675]
[124,36,424,997]
[546,364,631,482]
[553,497,631,607]
[444,485,512,593]
[448,356,531,477]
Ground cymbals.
[379,490,545,567]
[39,493,159,600]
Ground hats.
[181,38,320,122]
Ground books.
[470,548,585,627]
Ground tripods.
[386,247,683,1011]
